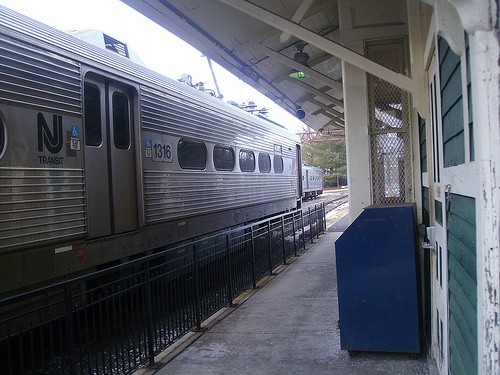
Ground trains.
[303,166,323,201]
[0,3,302,342]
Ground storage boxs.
[335,203,421,361]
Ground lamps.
[287,45,313,80]
